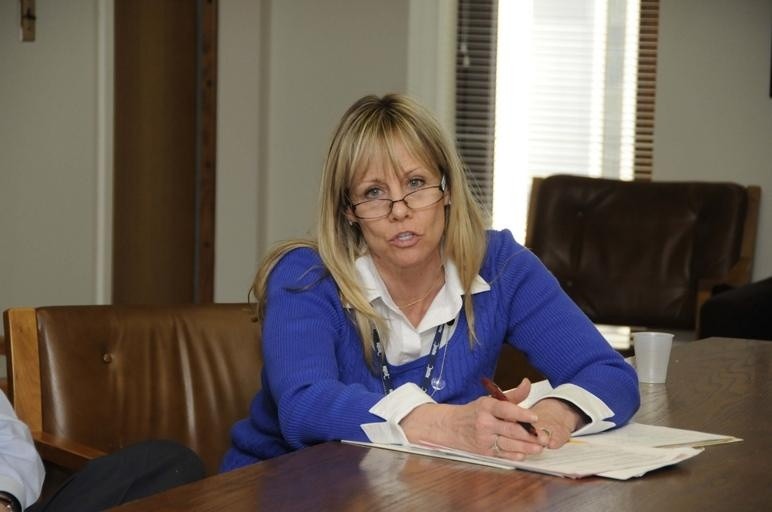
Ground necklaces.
[398,269,444,313]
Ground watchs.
[0,492,23,512]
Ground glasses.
[343,173,447,219]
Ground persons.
[1,381,206,510]
[217,92,642,475]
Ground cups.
[631,330,676,384]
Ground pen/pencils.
[480,374,538,437]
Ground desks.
[100,333,772,512]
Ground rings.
[539,427,553,440]
[489,434,505,453]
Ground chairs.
[2,303,268,474]
[525,172,760,342]
